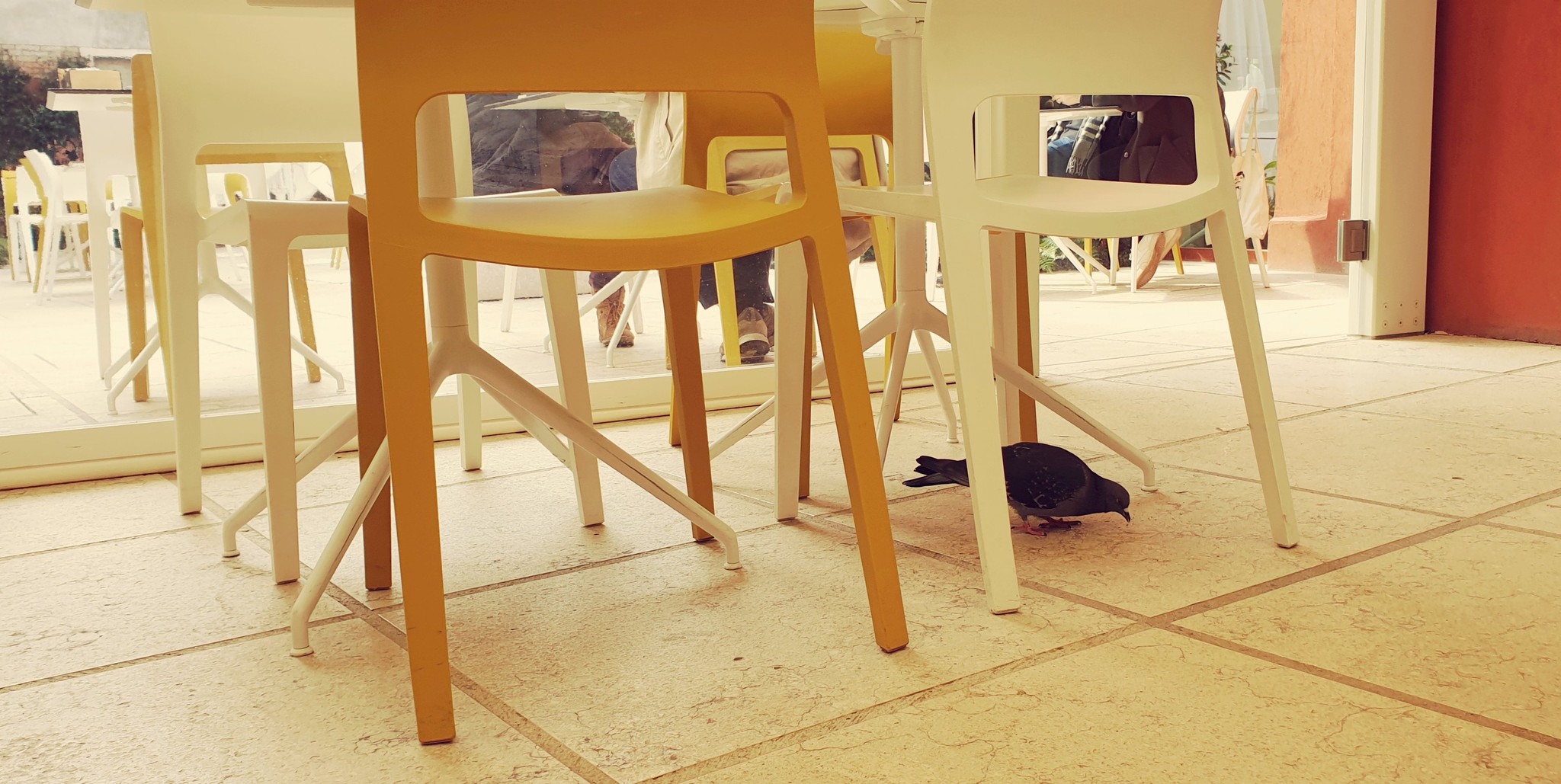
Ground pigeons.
[902,442,1130,537]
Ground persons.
[1038,95,1232,290]
[460,90,875,363]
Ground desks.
[708,0,1156,494]
[43,89,140,383]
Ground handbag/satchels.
[1204,87,1269,246]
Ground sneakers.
[761,304,774,348]
[720,307,769,363]
[1129,232,1166,289]
[592,285,634,348]
[1159,227,1182,263]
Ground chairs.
[0,0,1300,747]
